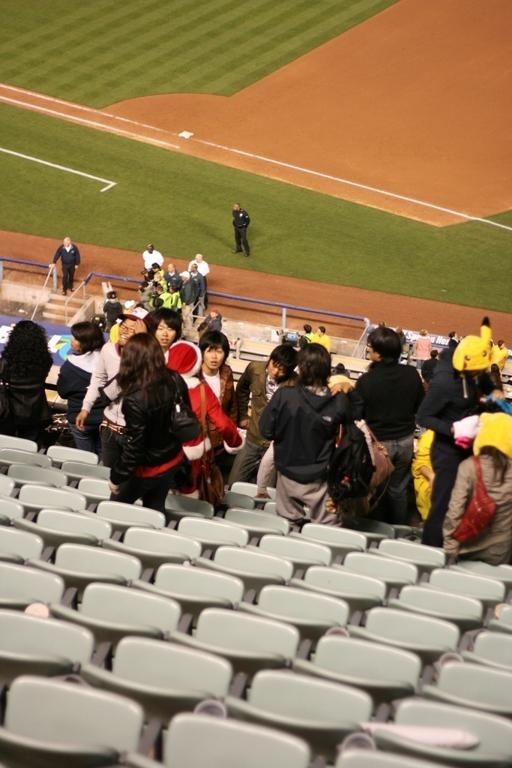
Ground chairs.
[0,432,512,766]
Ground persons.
[227,345,298,498]
[49,237,80,295]
[231,203,251,257]
[258,316,512,571]
[56,291,245,517]
[1,320,54,449]
[139,244,210,319]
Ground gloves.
[453,415,479,442]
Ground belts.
[101,421,123,435]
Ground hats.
[118,307,154,331]
[474,412,512,458]
[165,340,204,376]
[452,318,494,372]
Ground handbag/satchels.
[367,429,395,487]
[453,462,496,541]
[170,394,200,443]
[198,461,224,508]
[331,424,373,494]
